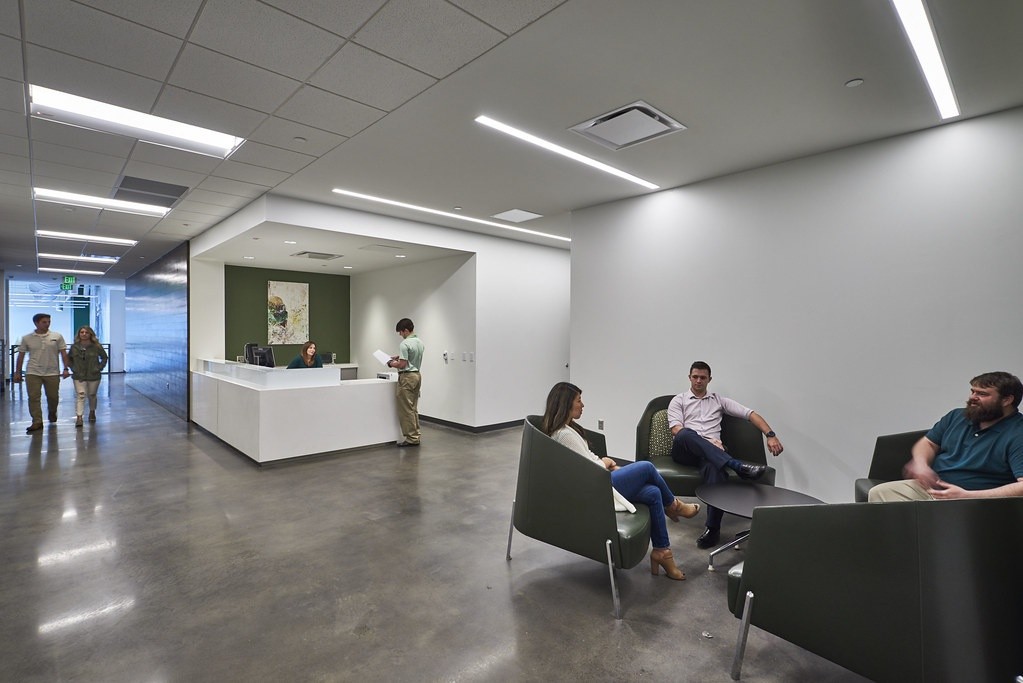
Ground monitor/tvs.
[244,343,258,363]
[252,346,275,367]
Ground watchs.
[766,431,775,438]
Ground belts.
[398,371,418,373]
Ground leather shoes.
[736,462,765,480]
[696,526,719,543]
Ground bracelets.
[64,368,68,370]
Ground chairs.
[636,396,776,496]
[854,429,930,501]
[507,415,650,618]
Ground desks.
[695,483,828,571]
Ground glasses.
[82,352,85,360]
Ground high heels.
[650,548,686,580]
[664,498,700,523]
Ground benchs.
[728,495,1023,683]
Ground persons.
[67,326,107,427]
[386,318,426,446]
[542,382,701,579]
[13,314,70,431]
[868,371,1023,503]
[668,361,783,545]
[286,341,323,369]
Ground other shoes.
[48,413,57,422]
[76,420,83,426]
[26,422,43,431]
[88,414,95,421]
[396,441,418,446]
